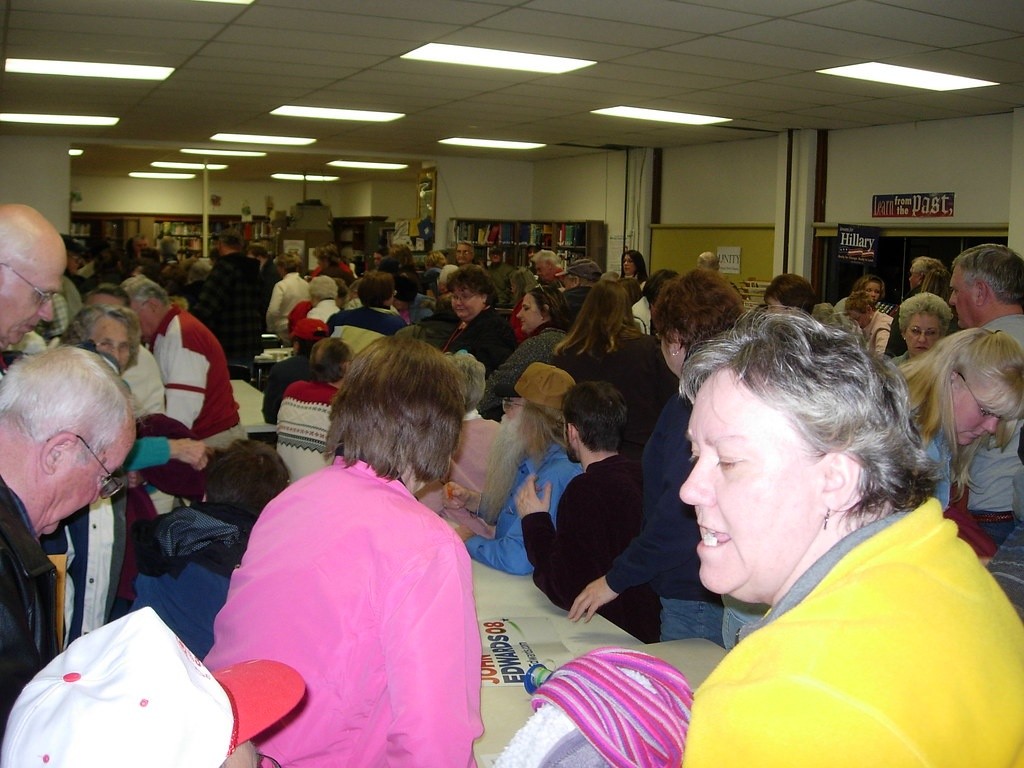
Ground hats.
[60,233,84,253]
[526,241,541,250]
[489,245,503,253]
[0,605,306,768]
[290,319,329,341]
[514,362,576,410]
[555,259,602,280]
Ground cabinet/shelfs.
[72,211,242,259]
[449,215,605,272]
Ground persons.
[0,606,309,767]
[676,304,1023,768]
[200,336,485,768]
[0,345,137,753]
[0,202,68,380]
[40,228,1023,652]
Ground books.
[64,221,279,260]
[452,221,586,266]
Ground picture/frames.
[416,166,438,243]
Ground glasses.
[957,371,1004,420]
[68,255,83,264]
[46,435,124,500]
[392,290,397,296]
[95,340,130,353]
[501,396,525,413]
[0,262,59,304]
[908,326,937,336]
[448,293,476,300]
